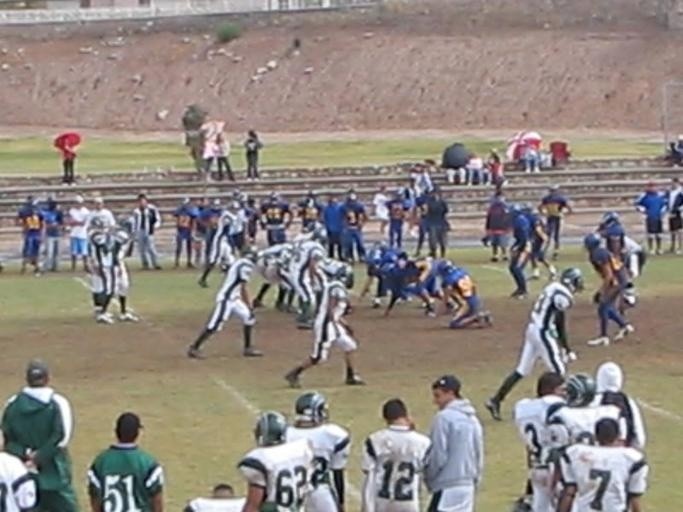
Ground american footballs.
[372,298,382,306]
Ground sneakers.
[284,371,299,388]
[243,347,263,356]
[477,311,485,327]
[90,217,109,228]
[586,338,609,347]
[96,315,113,324]
[345,375,366,385]
[484,397,503,421]
[486,311,493,325]
[187,348,206,358]
[614,325,633,341]
[119,312,136,321]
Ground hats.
[118,414,143,430]
[28,362,48,382]
[439,375,460,396]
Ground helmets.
[337,267,355,289]
[120,214,136,229]
[242,245,259,260]
[568,375,594,404]
[584,233,601,247]
[563,268,583,293]
[293,393,326,423]
[438,260,452,275]
[253,412,286,445]
[603,213,619,225]
[228,200,240,210]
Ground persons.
[257,192,298,246]
[559,417,645,512]
[174,197,197,271]
[0,429,37,512]
[296,187,327,234]
[372,184,393,235]
[282,264,369,389]
[668,135,683,166]
[68,193,91,275]
[60,129,76,188]
[186,243,266,358]
[513,371,572,512]
[508,201,536,301]
[582,229,635,345]
[378,250,435,317]
[86,412,165,512]
[484,189,509,263]
[195,199,239,288]
[85,217,113,306]
[523,141,542,173]
[636,178,667,256]
[254,240,285,311]
[428,186,450,257]
[385,189,411,251]
[199,200,229,267]
[412,186,438,257]
[202,129,217,184]
[285,224,328,329]
[422,375,485,512]
[361,398,436,512]
[444,166,465,185]
[663,173,683,255]
[38,192,63,272]
[337,191,372,266]
[402,176,424,237]
[182,484,248,512]
[398,254,459,314]
[214,129,237,182]
[409,161,431,196]
[94,215,141,325]
[537,183,576,261]
[487,147,505,185]
[603,224,638,325]
[600,210,646,278]
[126,191,163,270]
[15,197,44,275]
[320,196,346,264]
[86,196,119,239]
[548,374,629,512]
[236,410,317,512]
[585,360,646,450]
[465,151,484,185]
[0,359,77,512]
[244,129,265,183]
[192,193,212,222]
[520,205,559,283]
[434,257,497,330]
[283,390,351,512]
[239,193,267,243]
[362,240,399,311]
